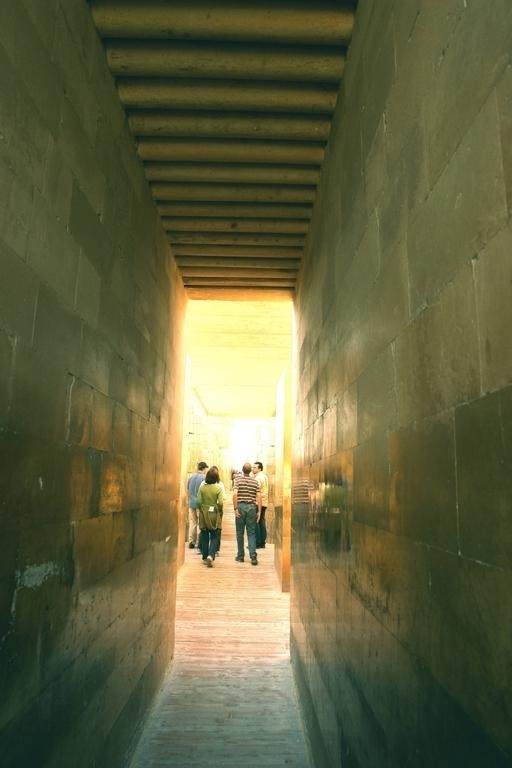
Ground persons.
[184,459,270,568]
